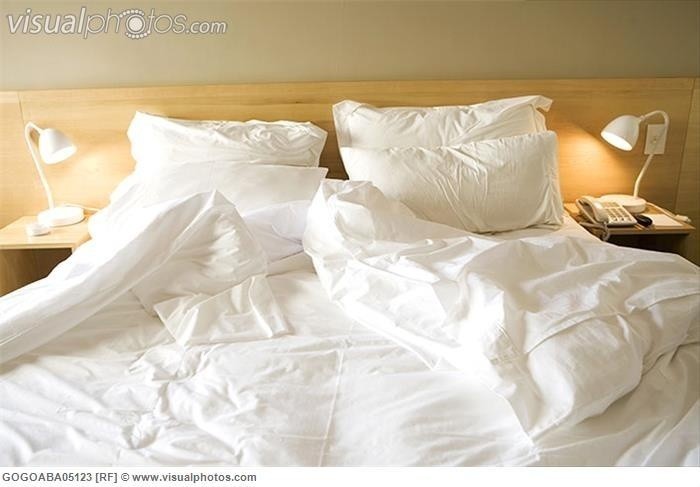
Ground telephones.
[576,194,638,227]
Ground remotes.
[635,214,652,226]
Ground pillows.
[339,129,564,234]
[332,94,554,148]
[126,110,329,207]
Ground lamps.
[600,110,670,214]
[24,123,84,228]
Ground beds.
[0,75,699,466]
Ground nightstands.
[0,215,92,297]
[562,202,696,263]
